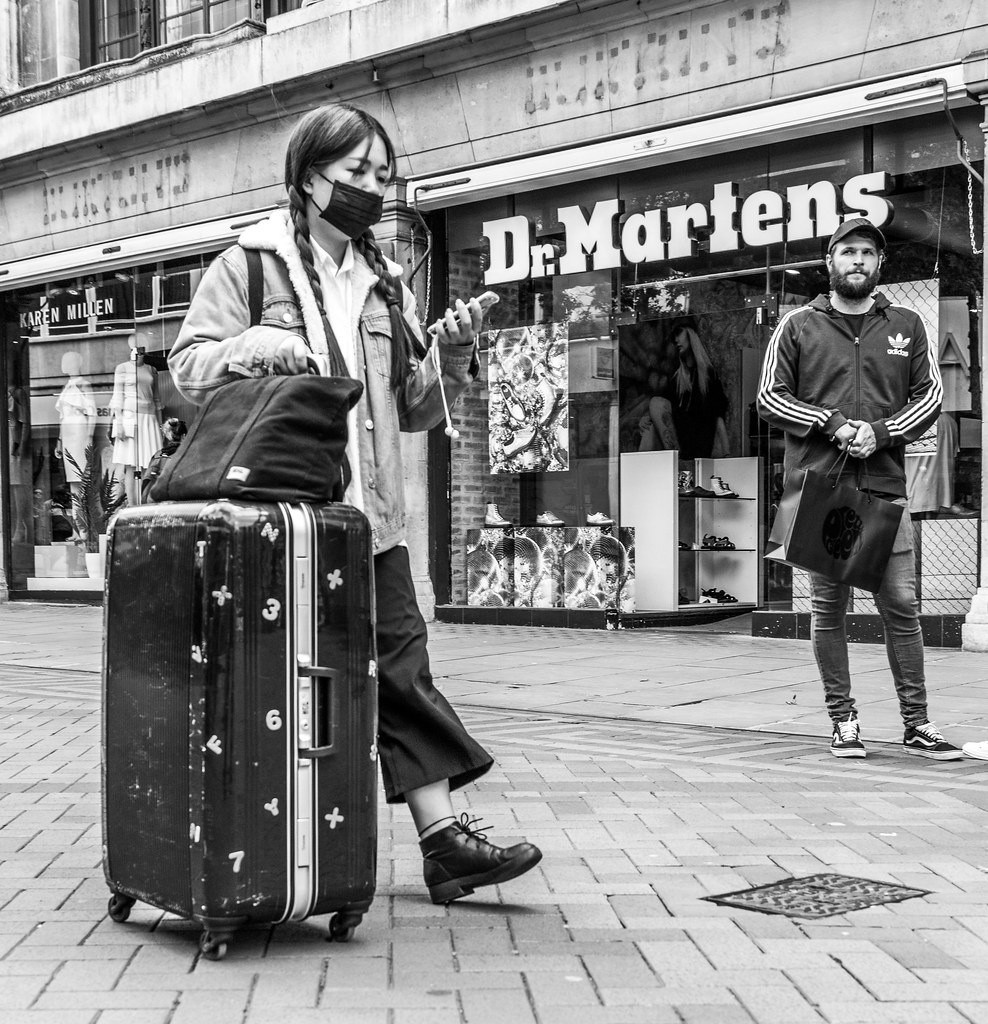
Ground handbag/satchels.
[763,439,905,594]
[150,375,365,501]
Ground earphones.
[445,426,460,439]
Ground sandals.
[679,541,692,550]
[701,534,735,549]
[699,587,738,603]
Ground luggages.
[101,353,377,961]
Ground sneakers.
[903,720,963,760]
[830,712,866,758]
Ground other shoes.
[587,511,616,526]
[65,528,89,542]
[679,587,690,604]
[678,486,716,497]
[962,741,988,760]
[536,513,565,527]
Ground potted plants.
[59,442,127,580]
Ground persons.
[173,104,542,906]
[638,308,731,458]
[22,488,52,546]
[8,333,165,551]
[756,218,965,761]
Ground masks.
[309,172,384,242]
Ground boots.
[484,503,513,528]
[709,475,740,499]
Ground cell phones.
[427,291,500,336]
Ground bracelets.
[725,454,731,456]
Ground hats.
[664,315,702,341]
[827,218,886,253]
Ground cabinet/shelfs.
[621,450,765,611]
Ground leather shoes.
[418,812,543,904]
